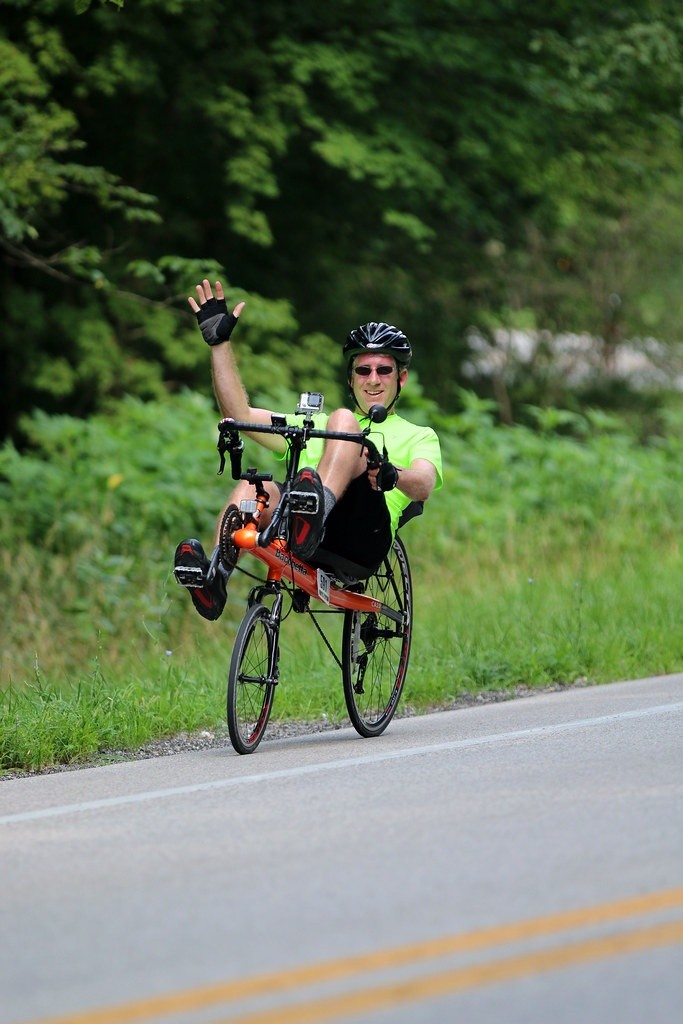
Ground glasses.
[353,366,397,376]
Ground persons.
[174,278,444,621]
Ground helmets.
[343,321,412,367]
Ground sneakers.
[174,539,227,620]
[290,467,325,556]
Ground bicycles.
[214,402,416,755]
